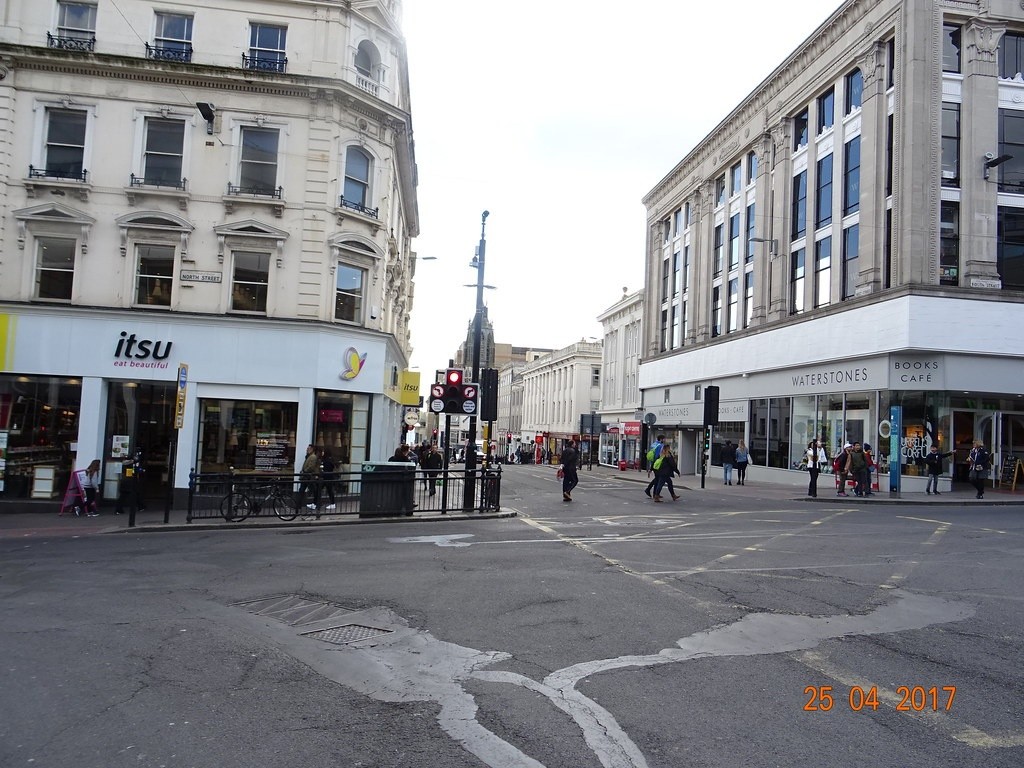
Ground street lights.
[464,210,497,511]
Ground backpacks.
[653,455,666,470]
[647,445,661,461]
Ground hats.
[844,444,852,449]
[864,443,872,451]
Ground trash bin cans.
[360,461,416,516]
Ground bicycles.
[220,476,298,523]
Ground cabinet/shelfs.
[7,445,61,477]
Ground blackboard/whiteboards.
[998,454,1024,485]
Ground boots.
[654,495,663,502]
[672,495,680,500]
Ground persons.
[75,460,100,517]
[561,440,578,502]
[924,444,956,494]
[510,445,553,464]
[720,440,736,485]
[806,442,823,496]
[835,438,874,497]
[736,440,749,486]
[115,453,144,514]
[644,434,665,498]
[294,444,336,510]
[387,440,442,509]
[654,445,681,502]
[452,447,465,465]
[966,439,990,499]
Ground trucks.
[463,439,485,463]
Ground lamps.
[748,238,778,255]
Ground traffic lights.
[491,445,496,449]
[535,436,543,443]
[433,429,438,440]
[508,434,511,443]
[704,429,710,438]
[445,369,461,412]
[704,440,709,450]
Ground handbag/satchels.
[747,453,752,466]
[969,471,977,480]
[817,448,827,463]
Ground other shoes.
[729,481,732,486]
[563,498,570,501]
[736,481,741,484]
[742,480,744,485]
[564,492,573,500]
[87,511,99,517]
[977,494,983,499]
[926,489,930,495]
[659,496,663,498]
[837,492,875,497]
[932,491,941,495]
[645,488,651,498]
[414,504,418,507]
[74,506,80,516]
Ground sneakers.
[326,504,336,509]
[307,503,316,510]
